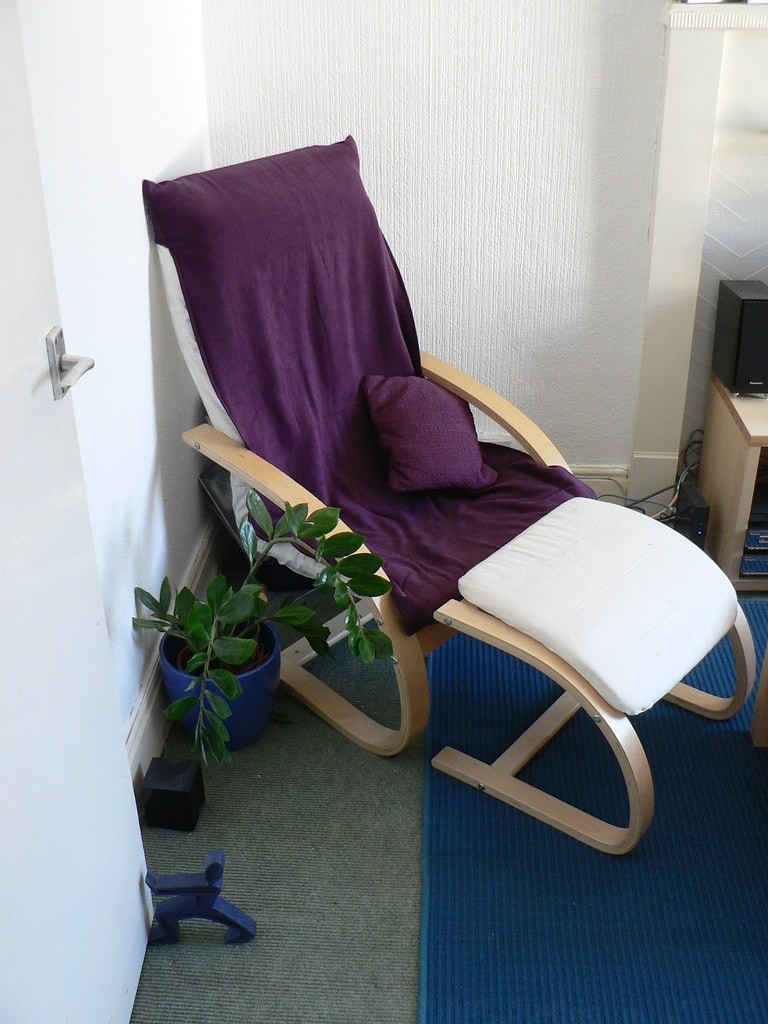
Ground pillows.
[364,375,498,494]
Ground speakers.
[711,280,768,398]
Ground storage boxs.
[141,757,206,832]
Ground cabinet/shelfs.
[697,374,766,592]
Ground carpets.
[421,595,768,1023]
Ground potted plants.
[131,488,395,769]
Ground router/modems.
[674,480,711,551]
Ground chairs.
[143,133,757,856]
